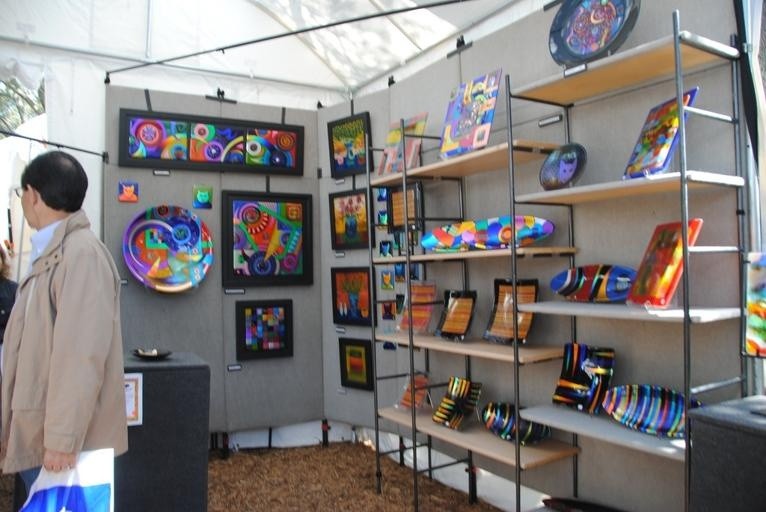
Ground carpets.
[1,442,503,511]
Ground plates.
[434,289,477,344]
[550,264,637,302]
[433,376,481,430]
[539,142,587,189]
[549,1,642,67]
[601,384,703,439]
[482,278,539,344]
[482,400,552,446]
[552,343,615,415]
[130,348,173,359]
[123,204,214,293]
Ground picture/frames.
[237,301,293,359]
[331,267,375,327]
[329,188,375,252]
[221,190,312,288]
[337,337,375,393]
[327,112,375,178]
[118,109,304,176]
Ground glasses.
[15,187,23,196]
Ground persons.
[0,150,129,496]
[0,245,19,345]
